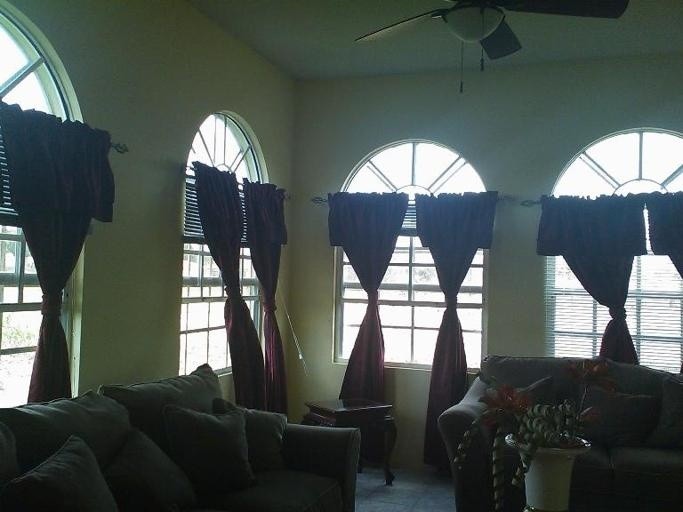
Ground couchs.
[0,364,362,511]
[436,354,683,512]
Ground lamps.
[444,0,505,43]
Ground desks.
[300,397,396,485]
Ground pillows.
[102,428,198,511]
[211,396,287,472]
[161,403,258,496]
[579,383,660,447]
[652,373,682,449]
[0,431,120,512]
[486,376,557,403]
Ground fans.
[354,0,628,62]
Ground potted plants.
[451,398,595,512]
